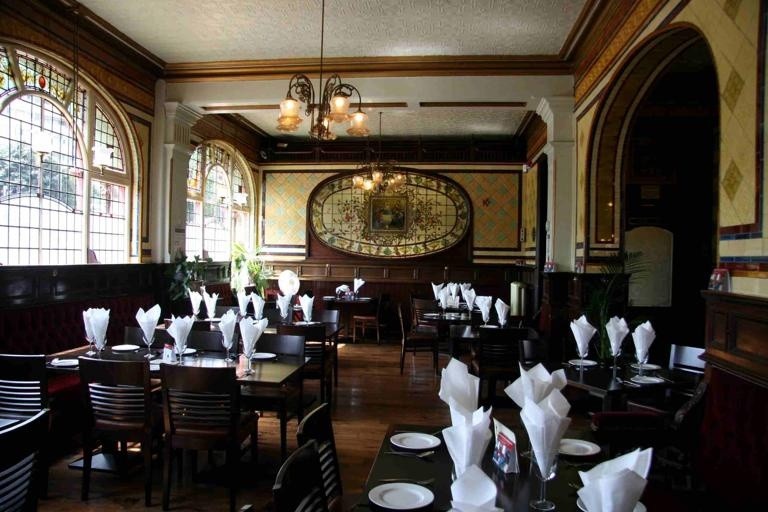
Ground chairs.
[272,440,327,512]
[668,345,707,399]
[408,290,417,333]
[77,355,199,508]
[254,333,305,456]
[234,323,262,418]
[398,303,440,376]
[159,363,259,512]
[0,353,49,500]
[185,331,239,353]
[276,322,327,419]
[125,327,174,349]
[297,401,359,512]
[353,293,382,345]
[312,310,339,342]
[245,302,292,322]
[0,407,40,512]
[164,319,211,331]
[209,306,240,319]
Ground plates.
[368,482,435,509]
[631,364,661,369]
[173,348,197,354]
[112,344,140,350]
[630,376,664,383]
[577,496,647,512]
[568,360,597,365]
[559,438,600,455]
[241,353,277,358]
[390,433,441,449]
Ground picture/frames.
[369,195,410,234]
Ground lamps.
[276,1,370,141]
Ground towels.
[439,358,481,413]
[633,320,656,362]
[277,294,292,318]
[476,296,492,321]
[432,283,444,300]
[83,311,93,340]
[460,283,472,300]
[240,316,268,357]
[438,287,447,309]
[442,396,492,479]
[167,315,195,352]
[495,298,509,325]
[450,283,460,299]
[570,314,597,357]
[90,309,110,344]
[336,285,350,294]
[447,464,502,512]
[189,289,202,315]
[135,304,161,343]
[579,446,652,511]
[447,282,453,295]
[466,289,476,311]
[521,389,571,478]
[354,278,365,293]
[219,309,236,348]
[250,293,265,320]
[237,291,251,317]
[505,362,567,408]
[606,315,629,354]
[299,294,314,322]
[204,292,218,318]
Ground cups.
[174,341,187,366]
[221,335,235,362]
[242,344,256,374]
[609,343,621,369]
[143,333,157,358]
[85,328,96,356]
[576,342,588,371]
[93,334,107,360]
[634,349,649,378]
[529,448,559,510]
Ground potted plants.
[167,245,213,301]
